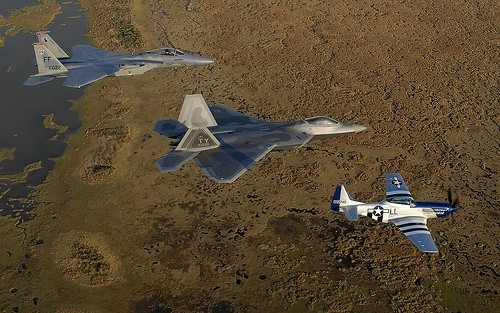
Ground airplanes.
[23,30,216,89]
[152,93,368,183]
[330,172,460,252]
